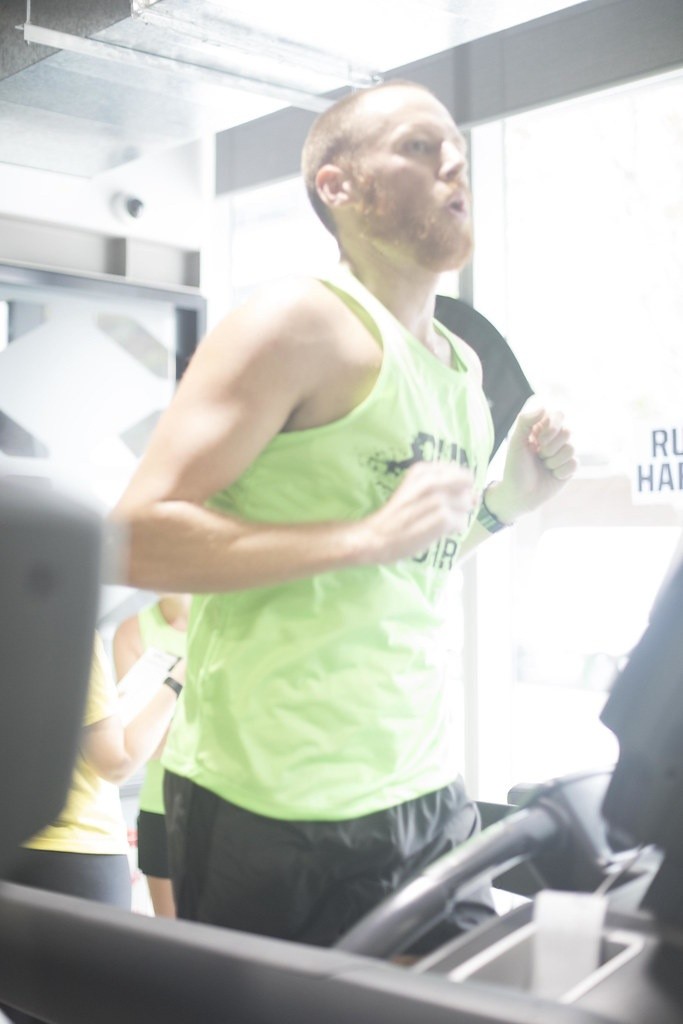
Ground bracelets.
[475,481,514,533]
[161,677,183,697]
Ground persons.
[100,78,575,947]
[0,607,188,916]
[112,591,188,921]
